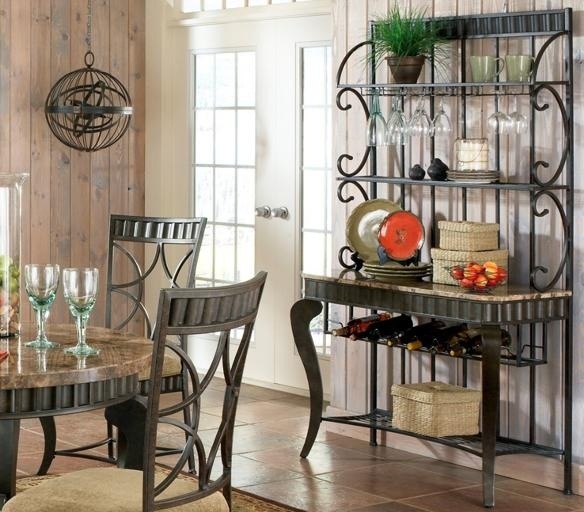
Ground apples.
[0,255,19,308]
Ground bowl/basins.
[446,265,509,293]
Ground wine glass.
[21,263,102,356]
[366,87,453,147]
[489,89,528,138]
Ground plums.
[449,262,506,292]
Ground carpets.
[1,461,309,512]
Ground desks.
[0,321,154,512]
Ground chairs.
[1,270,268,512]
[36,214,207,475]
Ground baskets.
[388,380,483,441]
[427,245,512,287]
[435,216,502,252]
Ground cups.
[469,55,535,85]
[455,137,489,173]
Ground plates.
[346,199,434,283]
[447,170,501,183]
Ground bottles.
[331,312,511,357]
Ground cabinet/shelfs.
[290,6,574,506]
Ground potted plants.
[359,0,456,85]
